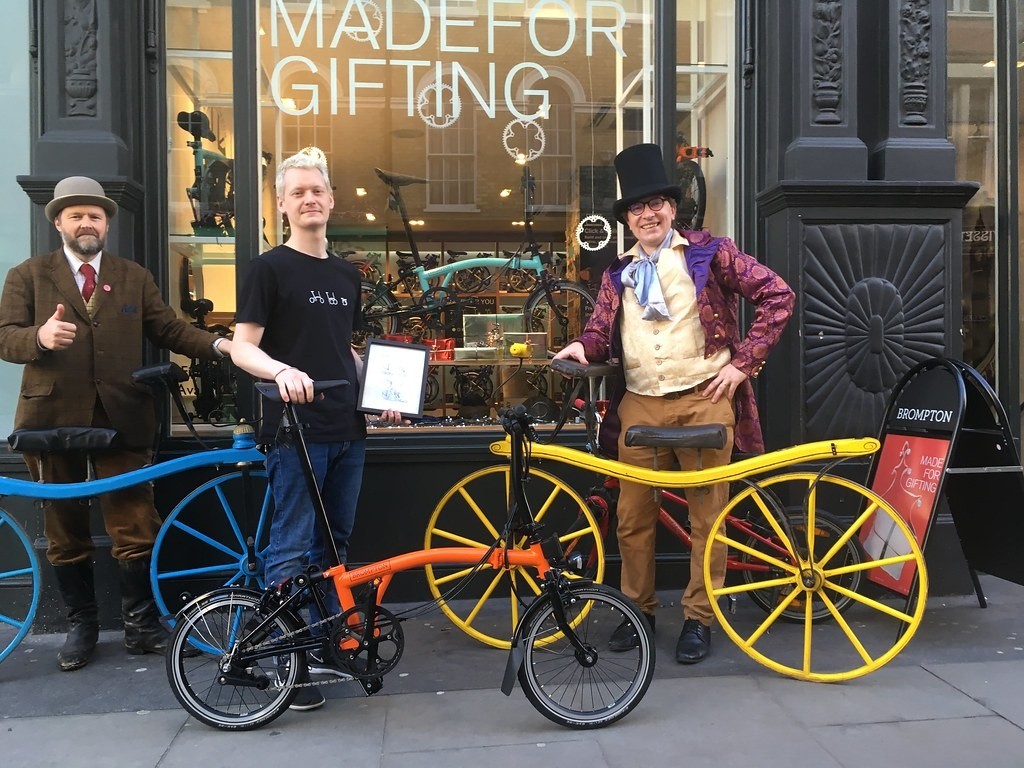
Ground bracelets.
[274,367,295,380]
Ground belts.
[663,377,716,400]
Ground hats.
[177,111,216,142]
[44,176,118,222]
[612,144,683,225]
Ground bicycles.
[176,109,273,231]
[671,131,714,233]
[332,247,567,337]
[353,163,600,344]
[162,380,656,737]
[182,294,240,416]
[422,330,928,683]
[1,361,280,665]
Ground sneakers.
[308,646,368,679]
[273,663,325,712]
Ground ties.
[80,265,95,301]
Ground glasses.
[624,197,670,215]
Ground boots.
[117,553,203,658]
[50,551,99,672]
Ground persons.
[0,176,232,670]
[229,146,410,712]
[549,185,796,665]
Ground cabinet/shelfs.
[170,235,569,428]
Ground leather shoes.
[674,618,710,664]
[610,613,657,652]
